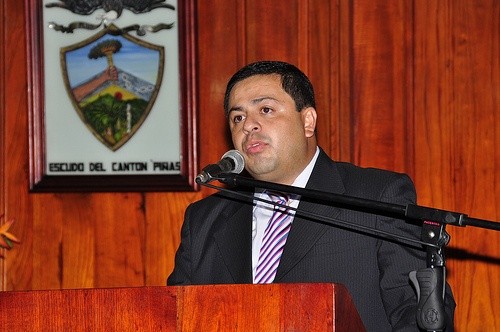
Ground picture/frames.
[22,0,203,195]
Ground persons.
[167,61,456,332]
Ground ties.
[254,193,291,285]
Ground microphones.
[196,150,245,183]
